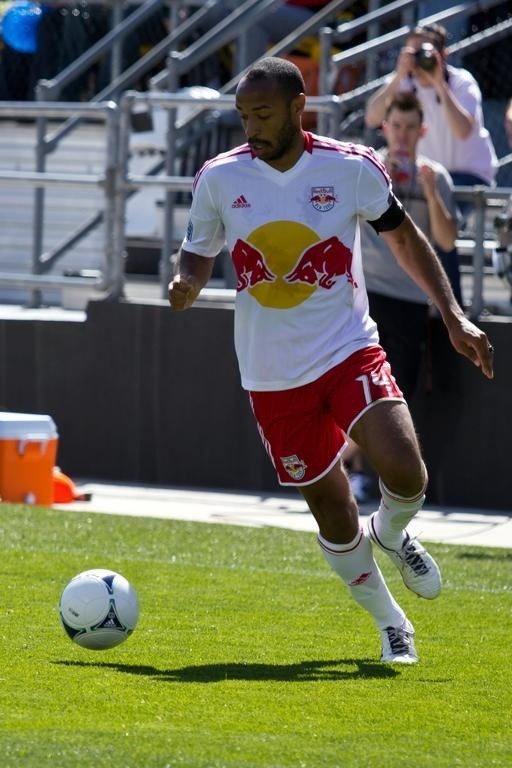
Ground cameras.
[388,155,414,176]
[410,41,438,70]
[491,213,511,229]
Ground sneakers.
[367,512,442,600]
[349,474,372,502]
[380,617,419,663]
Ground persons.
[363,21,501,313]
[1,1,399,132]
[342,86,466,501]
[489,97,511,307]
[164,57,494,666]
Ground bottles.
[388,145,410,182]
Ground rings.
[486,342,493,352]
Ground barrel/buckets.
[0,411,61,505]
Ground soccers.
[59,568,141,652]
